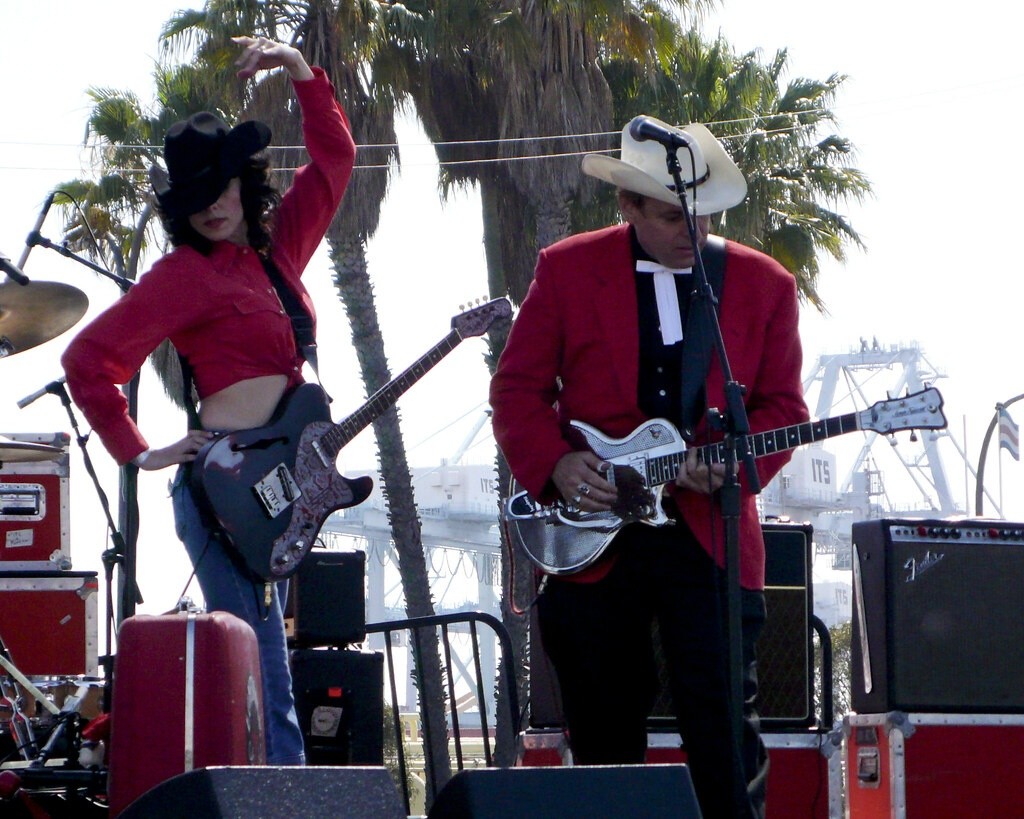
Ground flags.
[999,408,1020,461]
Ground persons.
[489,114,810,819]
[61,34,358,765]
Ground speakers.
[528,520,813,733]
[848,515,1024,715]
[118,764,409,819]
[427,763,703,819]
[283,550,365,648]
[290,647,384,767]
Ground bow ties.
[636,259,693,346]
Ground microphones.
[5,193,56,283]
[16,376,67,409]
[629,114,690,146]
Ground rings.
[572,496,581,505]
[576,482,590,495]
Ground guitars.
[188,293,523,579]
[507,383,949,577]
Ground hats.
[582,115,748,217]
[149,112,272,219]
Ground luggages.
[108,597,265,819]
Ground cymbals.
[0,280,91,357]
[0,434,65,464]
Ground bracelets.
[130,449,151,467]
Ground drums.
[22,668,111,734]
